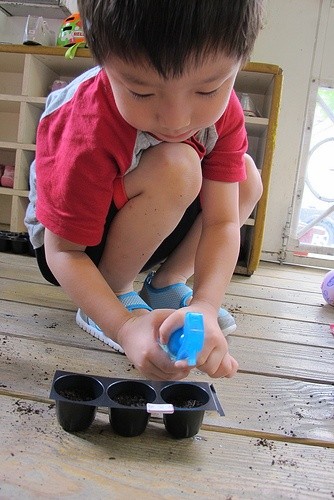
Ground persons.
[24,0,263,381]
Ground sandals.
[138,270,237,336]
[75,290,154,353]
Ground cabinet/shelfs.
[0,42,283,278]
